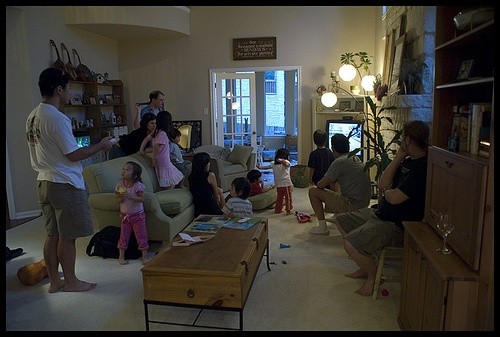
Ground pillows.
[226,144,254,168]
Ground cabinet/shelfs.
[396,220,480,331]
[423,7,494,274]
[65,78,126,143]
[312,96,367,162]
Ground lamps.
[318,51,376,161]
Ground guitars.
[49,39,71,80]
[60,42,77,80]
[73,48,92,81]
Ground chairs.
[371,245,405,301]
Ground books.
[185,214,231,233]
[222,215,261,230]
[448,101,491,159]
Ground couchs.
[183,143,257,194]
[80,152,195,241]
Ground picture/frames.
[339,101,351,111]
[387,33,406,95]
[383,29,394,91]
[355,101,363,112]
[232,37,277,61]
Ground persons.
[139,110,185,189]
[218,176,253,221]
[25,65,115,293]
[140,89,165,118]
[256,148,294,215]
[225,169,273,202]
[334,120,430,296]
[307,128,334,187]
[308,133,370,234]
[167,127,193,186]
[108,112,156,160]
[114,161,151,264]
[188,151,222,217]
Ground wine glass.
[437,213,454,254]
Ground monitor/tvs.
[325,120,364,155]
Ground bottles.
[109,112,116,123]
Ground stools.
[247,187,280,211]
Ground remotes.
[109,136,121,144]
